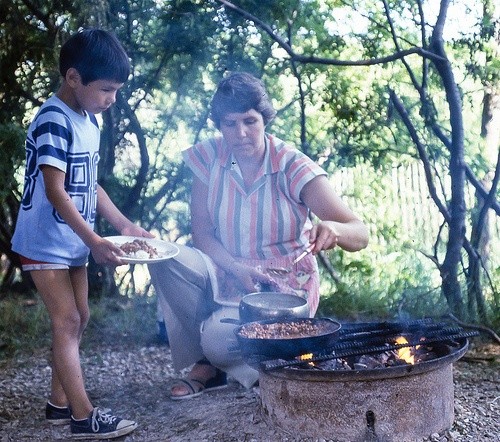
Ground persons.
[147,72,370,399]
[11,29,156,440]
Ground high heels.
[169,358,231,399]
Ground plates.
[101,236,180,264]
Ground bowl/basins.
[238,292,309,322]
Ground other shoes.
[45,399,73,425]
[70,407,139,440]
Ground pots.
[220,316,342,354]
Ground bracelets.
[226,258,235,277]
